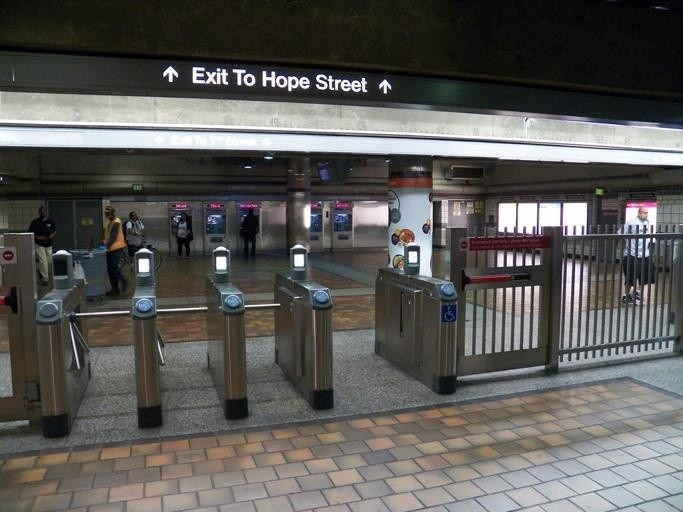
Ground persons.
[240,208,259,258]
[97,203,130,296]
[26,205,56,286]
[614,206,655,305]
[174,212,194,258]
[125,211,146,268]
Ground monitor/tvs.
[317,164,338,184]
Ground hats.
[104,206,115,213]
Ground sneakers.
[42,281,48,286]
[107,289,121,297]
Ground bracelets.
[135,230,137,235]
